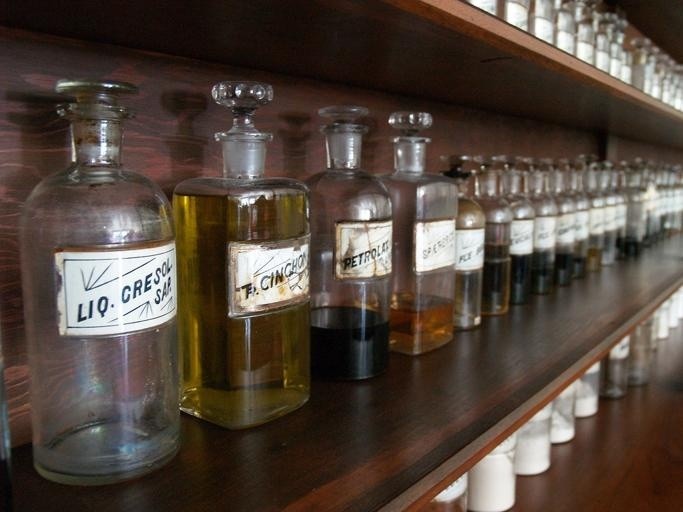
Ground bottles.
[455,0,683,114]
[381,104,456,355]
[417,283,683,512]
[438,152,683,329]
[173,77,315,430]
[306,99,397,389]
[22,77,183,479]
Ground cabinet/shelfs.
[0,0,683,511]
